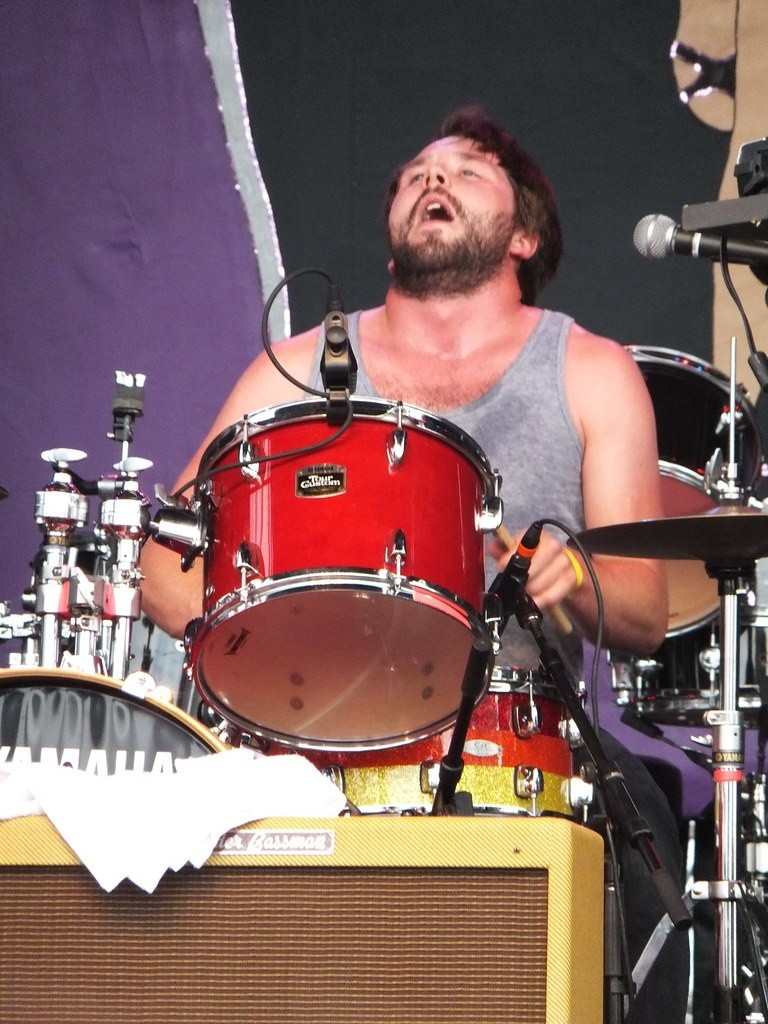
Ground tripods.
[622,558,768,1024]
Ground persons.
[140,108,685,1024]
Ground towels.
[0,742,353,899]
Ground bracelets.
[564,545,584,591]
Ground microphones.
[488,520,543,638]
[320,285,358,394]
[634,214,768,264]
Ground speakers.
[0,815,605,1024]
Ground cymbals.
[566,505,768,560]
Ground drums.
[0,667,230,774]
[193,396,496,753]
[633,603,768,729]
[621,344,764,640]
[230,665,576,820]
